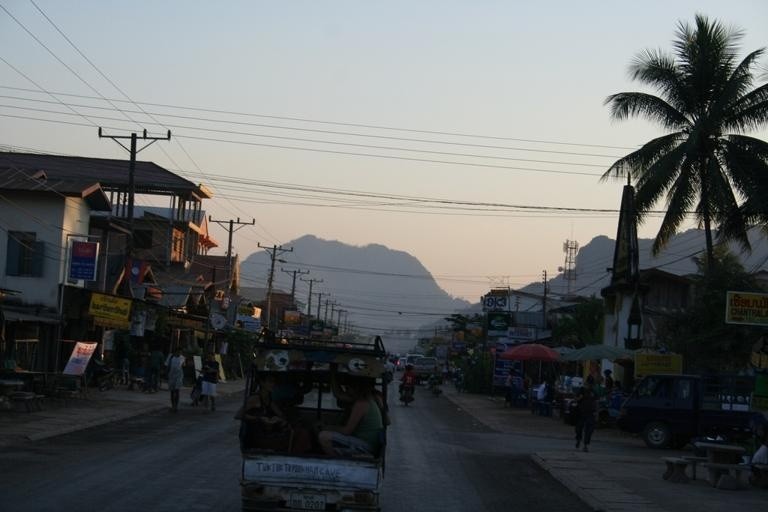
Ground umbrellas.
[500,344,628,383]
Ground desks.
[694,441,746,480]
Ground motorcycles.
[387,372,393,384]
[399,378,418,406]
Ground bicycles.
[190,369,205,402]
[144,372,162,393]
[99,370,123,392]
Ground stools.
[708,463,751,489]
[681,455,707,479]
[663,456,690,485]
[11,387,77,412]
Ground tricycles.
[238,329,387,511]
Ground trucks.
[398,354,443,384]
[617,375,765,450]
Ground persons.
[234,360,467,458]
[573,375,599,451]
[199,352,219,414]
[165,347,186,411]
[143,347,165,392]
[505,369,620,421]
